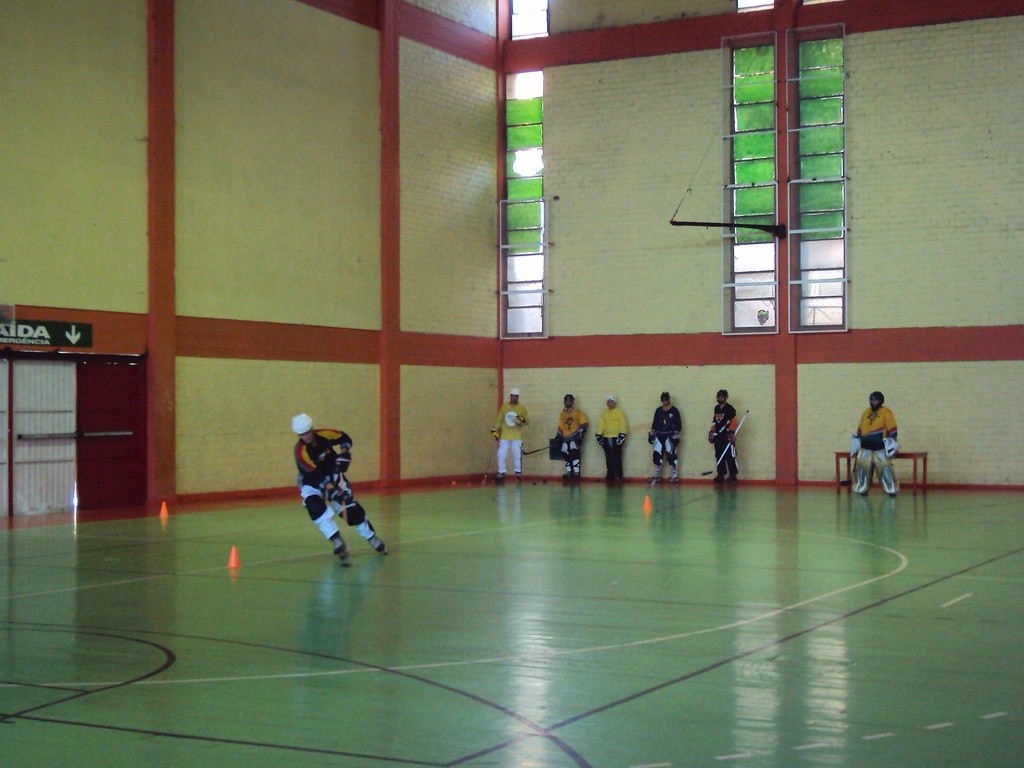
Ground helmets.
[510,388,519,395]
[716,389,728,404]
[661,391,670,401]
[291,414,313,435]
[608,395,616,402]
[870,391,885,411]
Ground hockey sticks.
[522,436,578,455]
[701,409,750,475]
[334,471,349,554]
[482,438,497,486]
[840,452,857,485]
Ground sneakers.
[330,531,347,559]
[367,535,388,554]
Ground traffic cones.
[228,546,239,568]
[644,495,652,512]
[160,502,169,518]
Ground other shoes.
[727,475,737,484]
[670,475,680,483]
[495,473,505,480]
[647,477,661,483]
[516,472,521,481]
[714,474,724,483]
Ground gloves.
[575,432,583,446]
[556,434,562,443]
[616,433,628,445]
[491,431,499,440]
[648,431,655,444]
[596,435,604,447]
[336,451,351,473]
[708,431,714,443]
[514,415,524,426]
[672,434,680,446]
[328,487,352,505]
[728,431,734,444]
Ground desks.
[832,450,930,497]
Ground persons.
[596,395,628,479]
[708,389,739,483]
[492,388,529,479]
[648,392,682,483]
[851,391,900,497]
[554,394,588,480]
[292,414,389,567]
